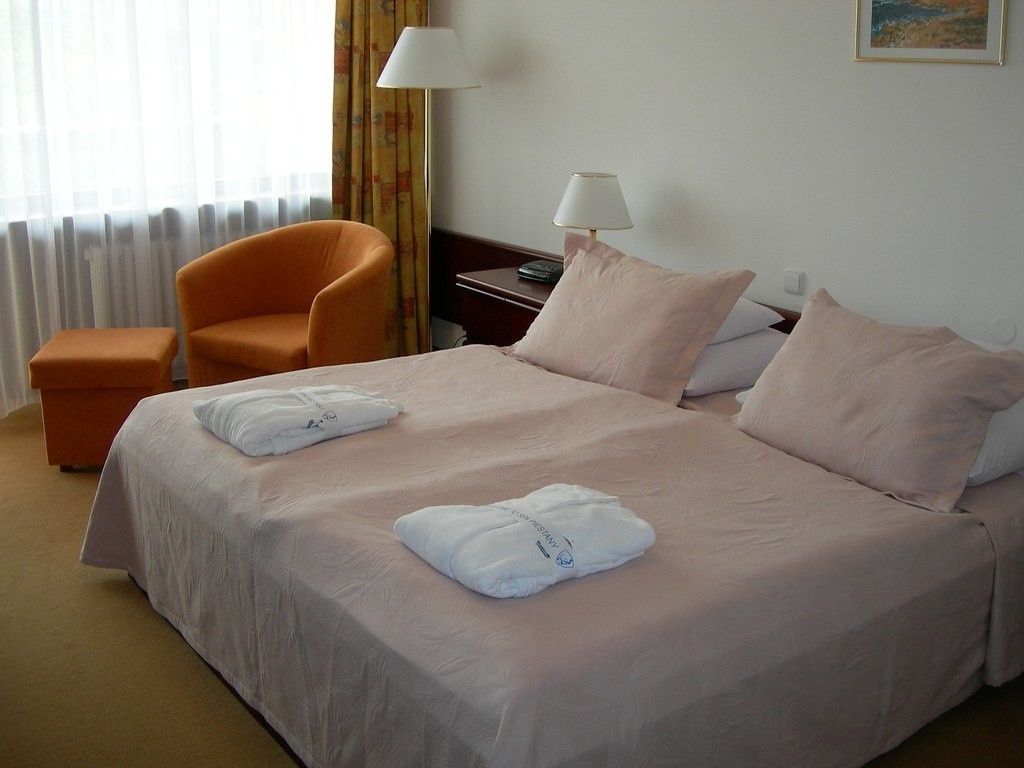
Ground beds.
[78,302,1023,768]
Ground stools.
[29,327,180,470]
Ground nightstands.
[456,266,558,346]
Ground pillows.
[489,232,757,406]
[707,296,785,346]
[968,397,1024,487]
[728,287,1024,513]
[682,327,789,397]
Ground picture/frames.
[854,0,1007,65]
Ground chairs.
[175,220,395,388]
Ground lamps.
[552,172,634,240]
[376,26,481,350]
